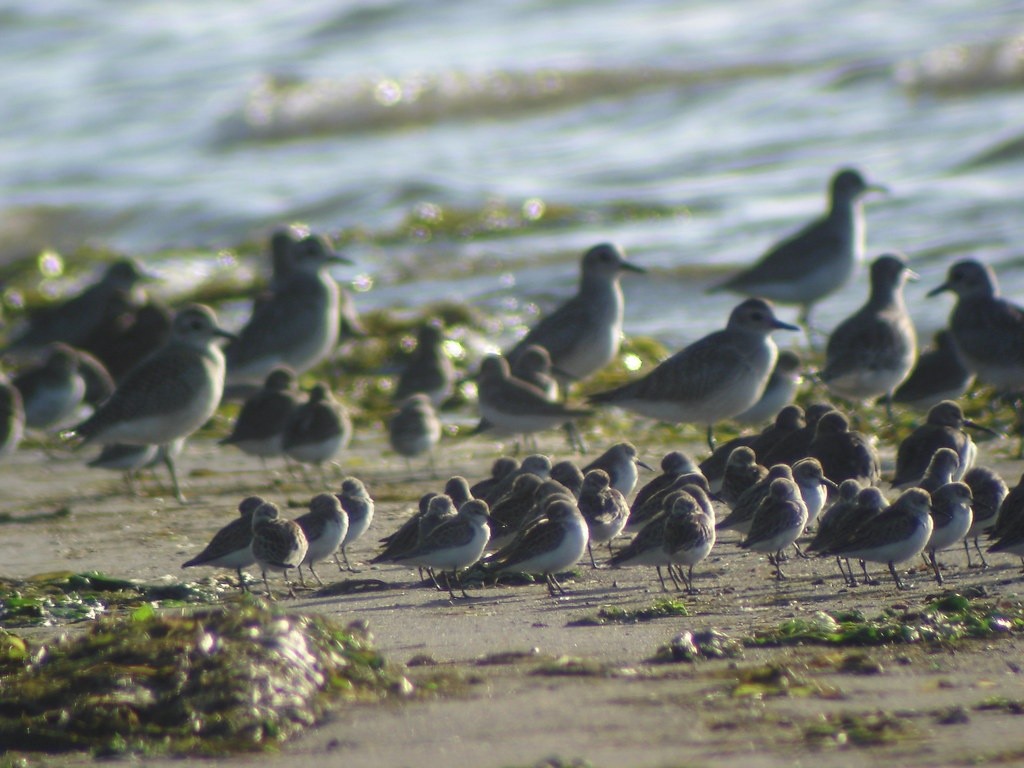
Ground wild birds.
[500,242,647,392]
[585,295,800,455]
[700,170,888,353]
[0,224,358,496]
[927,257,1024,410]
[808,255,923,426]
[182,347,1024,603]
[393,322,455,404]
